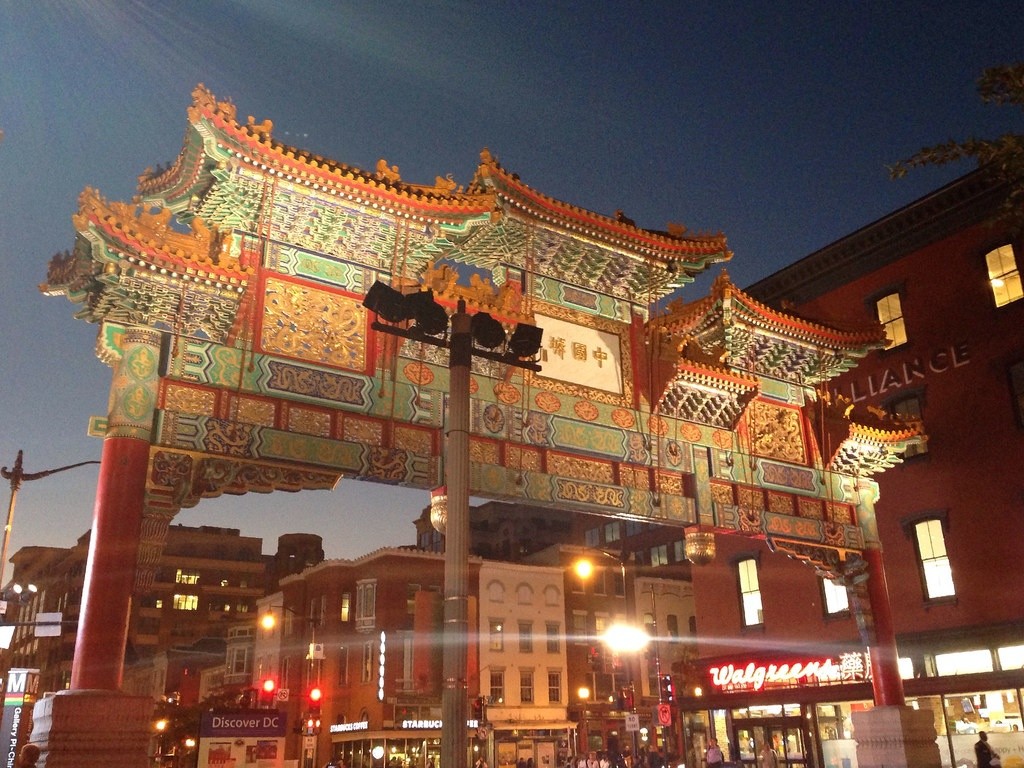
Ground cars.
[954,720,991,735]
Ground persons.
[974,730,1002,768]
[707,738,724,768]
[476,756,488,768]
[518,757,535,768]
[1012,724,1019,732]
[14,744,41,768]
[567,746,664,768]
[995,720,1002,724]
[323,757,347,768]
[388,756,403,768]
[958,717,978,734]
[738,732,780,768]
[426,757,436,768]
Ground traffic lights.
[308,682,321,716]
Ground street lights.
[261,679,275,708]
[574,545,640,768]
[361,279,544,768]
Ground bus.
[989,713,1024,732]
